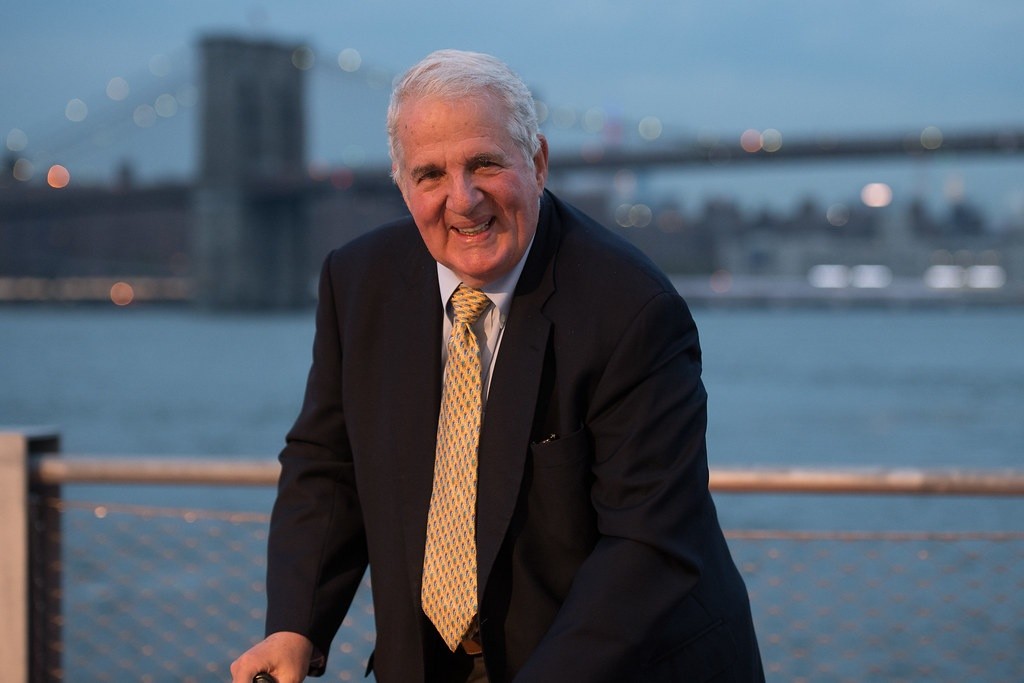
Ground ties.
[419,286,493,655]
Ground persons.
[228,50,766,683]
